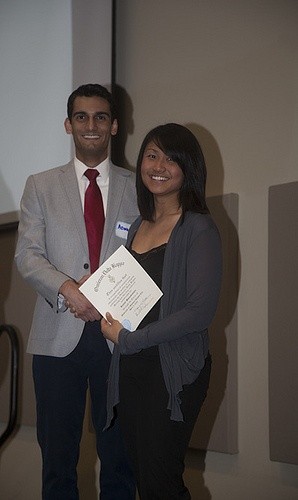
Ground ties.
[83,168,105,278]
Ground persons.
[65,122,223,500]
[13,83,141,500]
